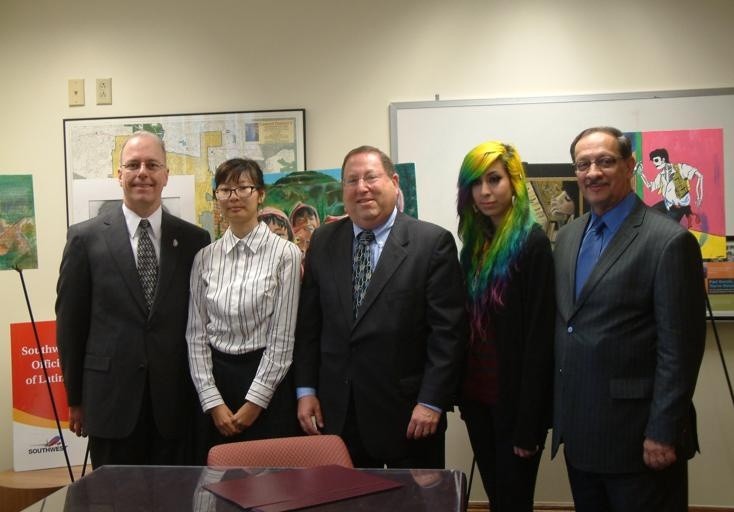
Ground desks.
[20,465,467,511]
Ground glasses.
[119,161,161,171]
[215,186,257,200]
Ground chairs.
[206,435,354,468]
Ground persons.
[255,205,295,242]
[55,130,212,475]
[550,127,707,511]
[289,199,320,230]
[457,141,559,511]
[185,157,304,464]
[551,183,579,216]
[295,147,463,467]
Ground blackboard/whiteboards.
[388,87,734,324]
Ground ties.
[137,219,158,311]
[352,230,375,322]
[575,217,604,302]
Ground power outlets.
[95,77,113,105]
